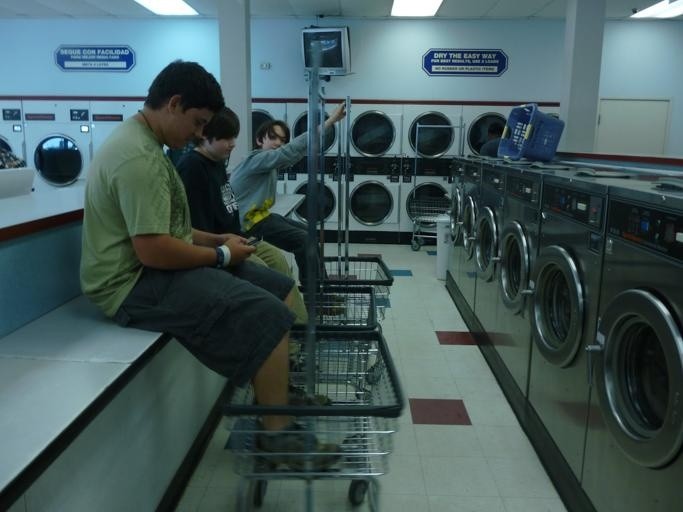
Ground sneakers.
[255,422,318,445]
[260,434,342,471]
[309,295,347,317]
[286,386,331,405]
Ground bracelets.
[213,245,231,270]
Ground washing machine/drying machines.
[249,96,341,235]
[347,96,462,241]
[442,154,682,511]
[20,98,91,191]
[88,99,169,182]
[462,97,563,243]
[0,97,27,170]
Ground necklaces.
[135,108,154,133]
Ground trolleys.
[221,43,406,512]
[407,121,467,253]
[288,86,393,386]
[320,98,395,319]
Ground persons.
[76,58,346,474]
[479,122,504,157]
[227,100,353,314]
[172,105,323,377]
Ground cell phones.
[249,238,263,246]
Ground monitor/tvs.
[302,26,351,75]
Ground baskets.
[495,104,565,162]
